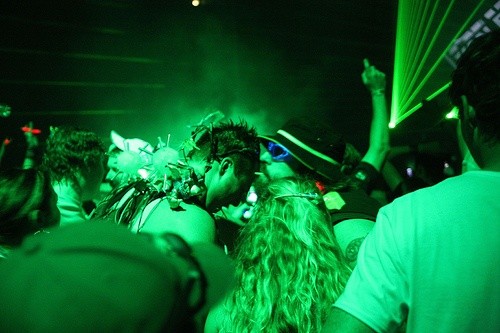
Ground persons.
[342,57,393,194]
[318,29,499,333]
[0,112,482,333]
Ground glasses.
[267,140,291,162]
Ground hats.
[256,116,346,182]
[0,221,192,333]
[110,129,153,155]
